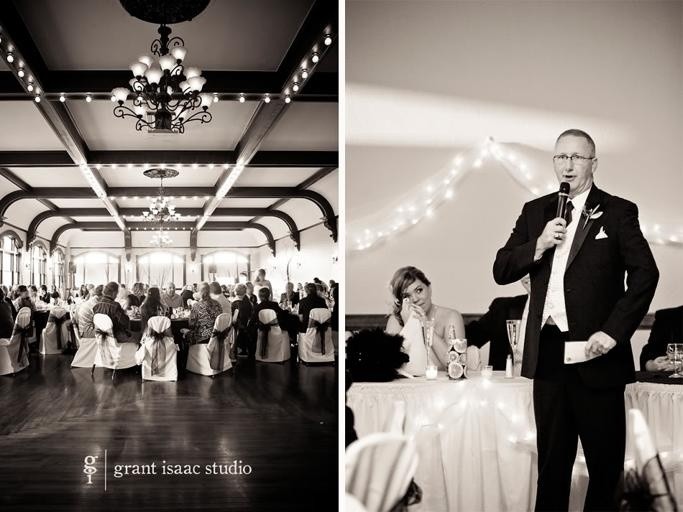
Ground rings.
[554,232,559,239]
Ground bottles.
[505,355,513,378]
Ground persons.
[0,268,339,375]
[639,305,682,372]
[384,265,465,377]
[492,129,660,512]
[464,272,532,372]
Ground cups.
[480,366,493,381]
[425,366,437,380]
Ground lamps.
[142,169,181,224]
[151,228,172,248]
[213,34,332,104]
[7,44,94,103]
[110,1,217,134]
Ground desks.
[346,370,590,511]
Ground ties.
[563,201,574,228]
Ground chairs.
[346,431,423,511]
[0,293,339,382]
[621,408,678,512]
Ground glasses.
[552,154,593,165]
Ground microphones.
[554,182,570,240]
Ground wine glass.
[666,343,683,379]
[424,320,435,365]
[507,319,522,378]
